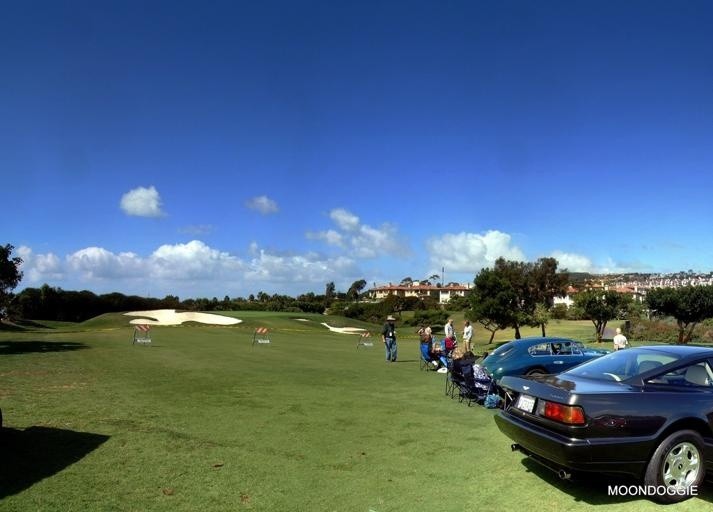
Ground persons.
[381,318,397,362]
[418,319,474,399]
[613,328,629,350]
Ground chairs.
[419,341,497,407]
[637,360,709,387]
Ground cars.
[478,336,713,504]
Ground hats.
[616,328,621,333]
[387,316,396,321]
